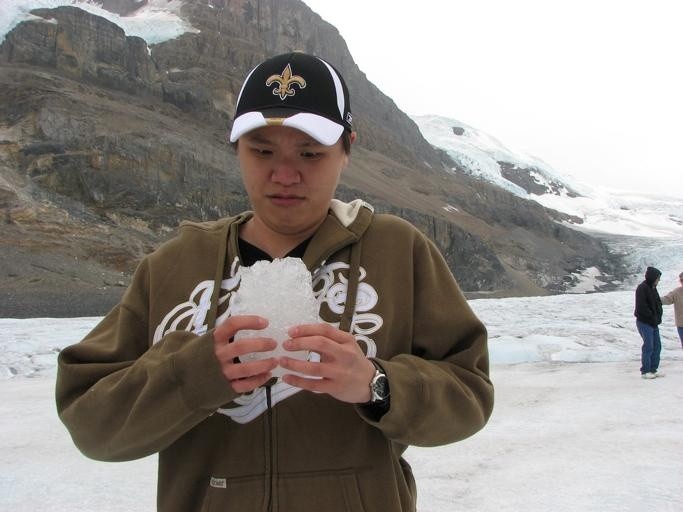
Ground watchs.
[370,359,390,409]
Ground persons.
[55,52,494,512]
[660,272,683,346]
[635,267,665,379]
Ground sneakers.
[641,370,666,380]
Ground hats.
[229,50,351,145]
